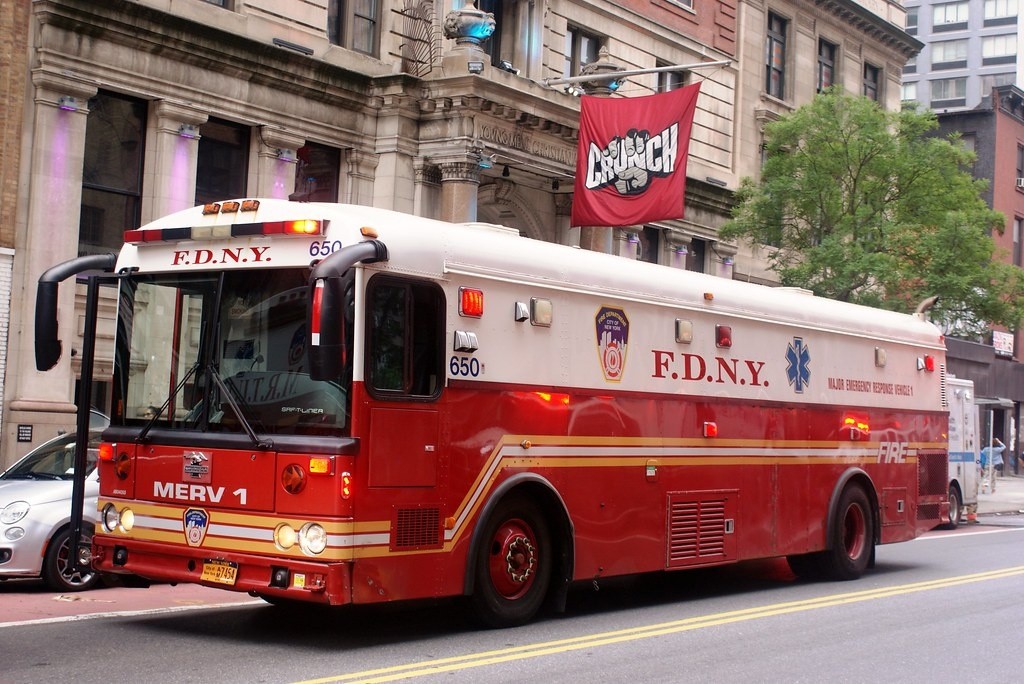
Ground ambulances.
[221,286,347,426]
[34,194,949,626]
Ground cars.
[0,426,108,592]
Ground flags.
[569,82,702,230]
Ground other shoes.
[991,488,995,493]
[982,487,985,493]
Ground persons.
[982,437,1007,494]
[959,464,986,523]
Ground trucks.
[932,373,979,529]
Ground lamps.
[468,62,484,74]
[565,86,578,96]
[674,246,688,254]
[722,256,733,264]
[497,59,520,75]
[553,181,559,190]
[502,166,510,177]
[627,232,639,243]
[478,154,494,169]
